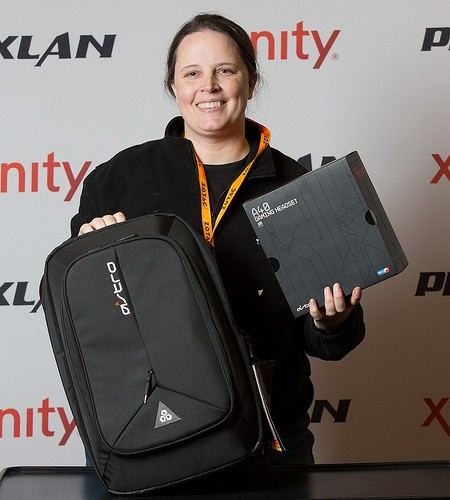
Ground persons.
[70,13,365,468]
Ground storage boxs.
[242,151,409,320]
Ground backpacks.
[39,213,270,495]
